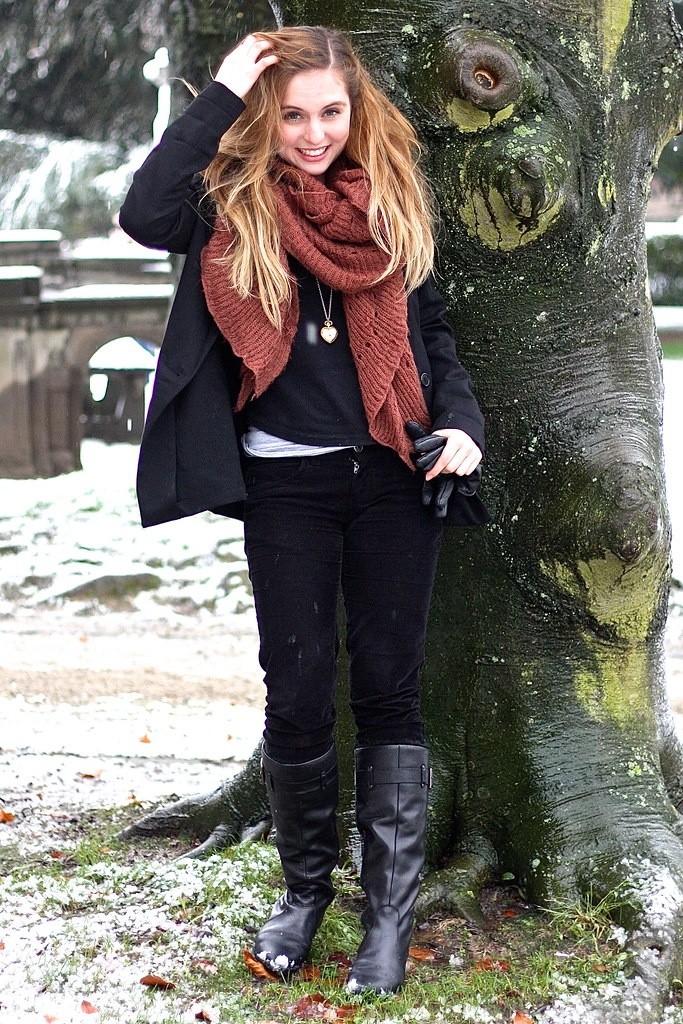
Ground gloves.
[404,420,481,519]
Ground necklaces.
[315,276,339,344]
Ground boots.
[253,736,340,977]
[345,745,435,995]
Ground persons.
[119,26,487,1001]
